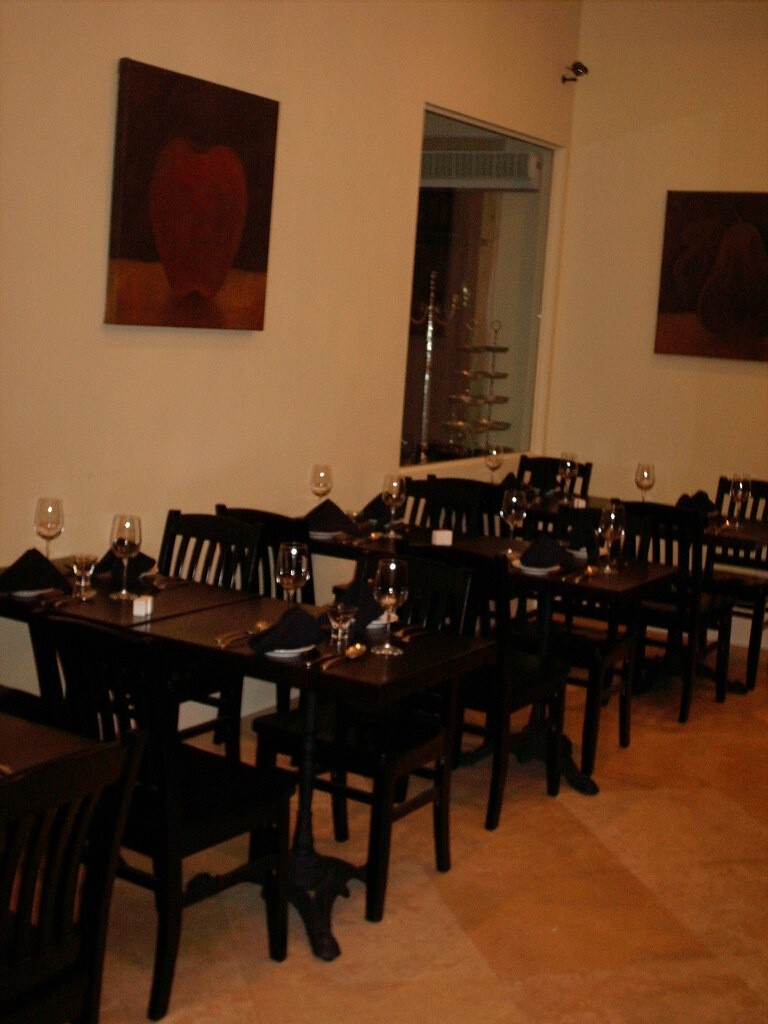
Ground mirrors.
[400,100,554,466]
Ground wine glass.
[557,452,578,505]
[729,472,751,529]
[382,474,406,538]
[370,558,408,656]
[635,463,655,502]
[597,504,625,576]
[34,498,64,560]
[275,542,311,609]
[500,489,527,555]
[109,515,141,600]
[310,464,333,505]
[485,445,504,484]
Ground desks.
[506,493,768,704]
[310,526,676,782]
[1,555,492,924]
[1,713,94,783]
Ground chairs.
[0,456,767,1024]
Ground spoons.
[320,643,366,671]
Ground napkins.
[495,474,525,492]
[92,538,156,579]
[352,492,400,526]
[250,604,326,657]
[517,533,578,570]
[669,494,691,521]
[677,491,719,522]
[0,547,74,595]
[299,499,366,538]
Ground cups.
[70,554,98,601]
[325,604,359,656]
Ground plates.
[366,610,398,629]
[264,643,315,658]
[309,531,341,540]
[511,559,560,576]
[565,547,609,559]
[12,587,54,598]
[369,518,403,528]
[100,567,159,583]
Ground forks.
[217,621,271,648]
